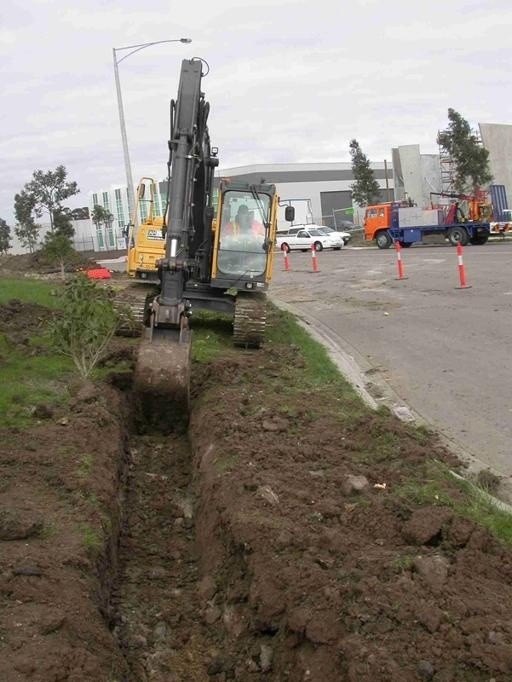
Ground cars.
[276,225,352,253]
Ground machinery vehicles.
[112,56,295,434]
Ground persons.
[223,204,265,239]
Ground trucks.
[363,190,495,249]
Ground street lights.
[112,38,192,218]
